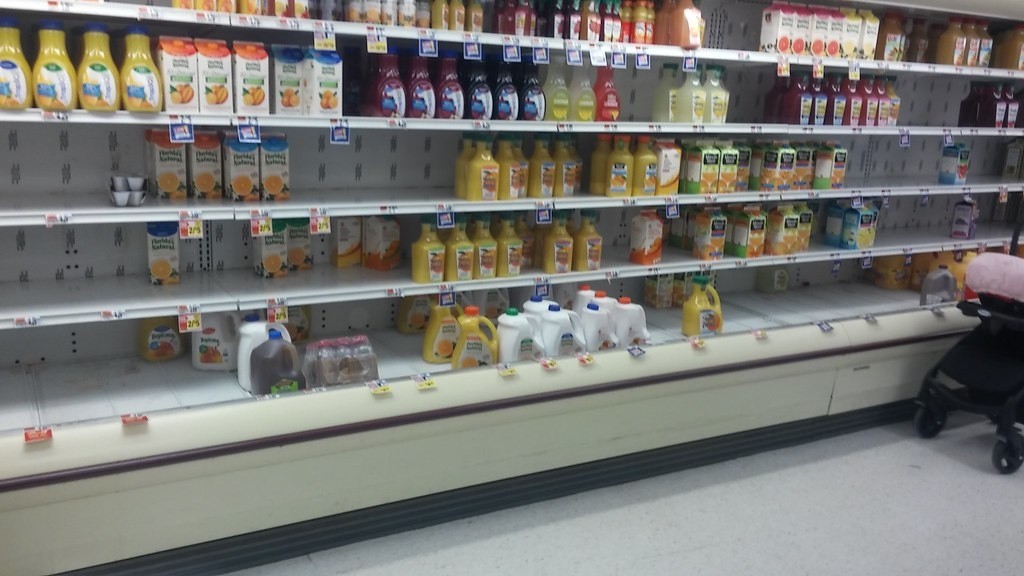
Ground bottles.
[651,62,730,125]
[453,133,658,202]
[395,292,652,372]
[347,0,484,33]
[874,9,1024,71]
[411,209,603,285]
[138,316,379,396]
[487,0,706,49]
[681,276,722,341]
[0,14,163,113]
[920,264,957,308]
[762,69,900,127]
[346,41,623,124]
[957,79,1024,129]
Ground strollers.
[908,200,1024,474]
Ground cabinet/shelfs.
[0,0,1024,576]
